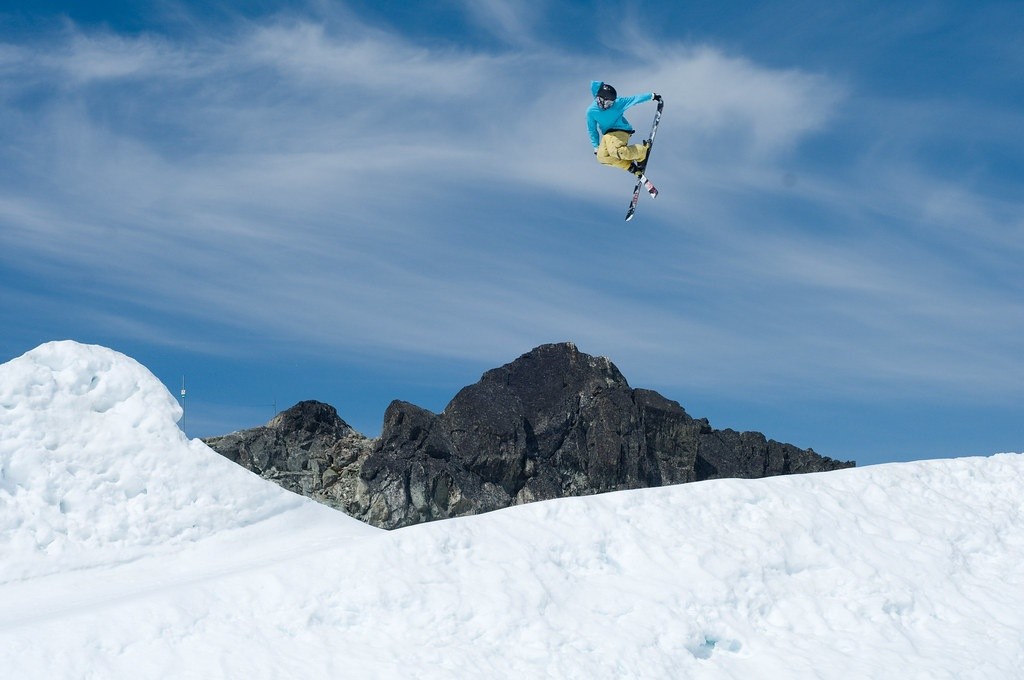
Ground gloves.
[653,93,661,103]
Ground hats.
[597,85,617,101]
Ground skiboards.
[624,99,665,222]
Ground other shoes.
[628,159,646,174]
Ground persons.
[585,84,661,178]
